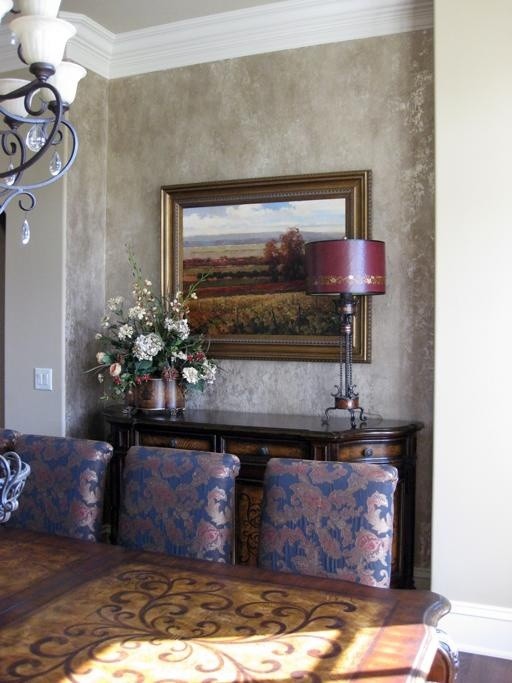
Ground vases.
[131,374,185,413]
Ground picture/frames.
[161,170,378,364]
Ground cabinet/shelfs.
[101,400,427,594]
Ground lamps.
[1,0,87,232]
[301,240,387,426]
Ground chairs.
[1,428,17,483]
[256,456,398,591]
[114,444,241,570]
[8,433,114,543]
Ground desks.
[0,519,466,683]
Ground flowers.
[75,242,220,411]
